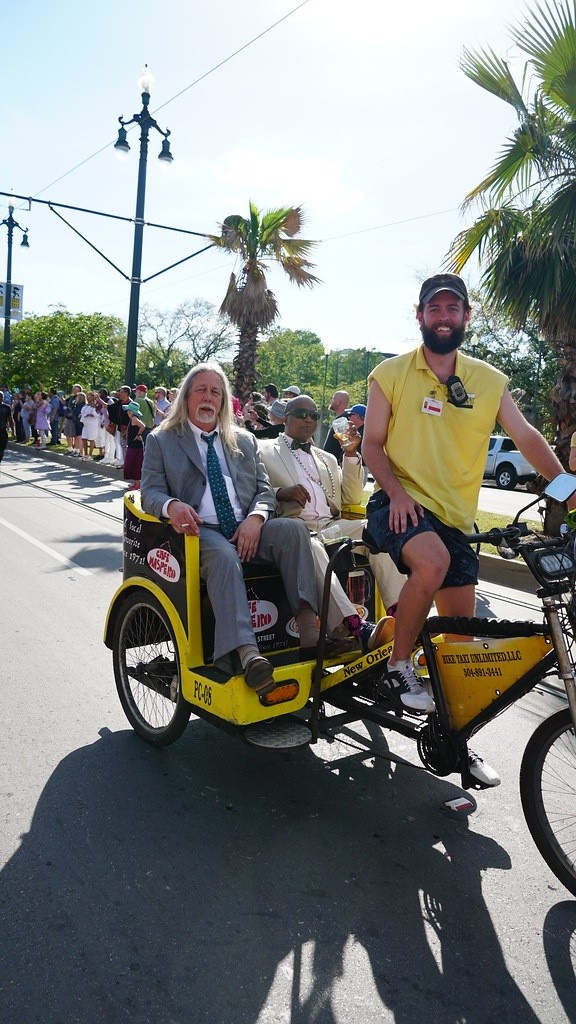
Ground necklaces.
[282,435,335,500]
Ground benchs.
[124,489,388,670]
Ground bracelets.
[137,434,141,436]
[156,409,160,412]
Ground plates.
[285,603,369,638]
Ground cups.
[348,572,365,607]
[38,438,40,446]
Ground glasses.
[350,413,357,418]
[286,392,291,395]
[286,407,320,421]
[154,392,161,395]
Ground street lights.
[364,343,373,404]
[0,188,31,353]
[321,346,331,413]
[167,359,172,389]
[112,62,174,391]
[536,329,548,386]
[148,360,154,388]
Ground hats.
[131,385,148,393]
[248,404,270,422]
[419,273,467,308]
[344,404,367,418]
[266,400,287,419]
[122,402,143,416]
[282,386,300,396]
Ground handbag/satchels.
[106,423,116,434]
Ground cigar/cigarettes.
[180,522,196,528]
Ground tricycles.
[102,471,576,896]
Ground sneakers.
[382,655,436,711]
[465,744,501,786]
[355,615,395,655]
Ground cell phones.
[446,375,468,407]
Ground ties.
[201,432,238,546]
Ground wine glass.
[332,417,354,447]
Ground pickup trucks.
[482,436,539,490]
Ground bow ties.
[290,440,311,453]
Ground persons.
[323,390,350,465]
[79,385,134,465]
[131,385,179,447]
[0,385,52,464]
[569,431,576,471]
[140,362,356,689]
[283,385,300,398]
[361,274,576,785]
[344,404,367,487]
[123,402,146,490]
[49,383,88,458]
[233,384,287,439]
[255,396,409,653]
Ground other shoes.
[128,483,140,491]
[243,655,275,696]
[6,435,61,450]
[63,448,125,469]
[298,636,352,660]
